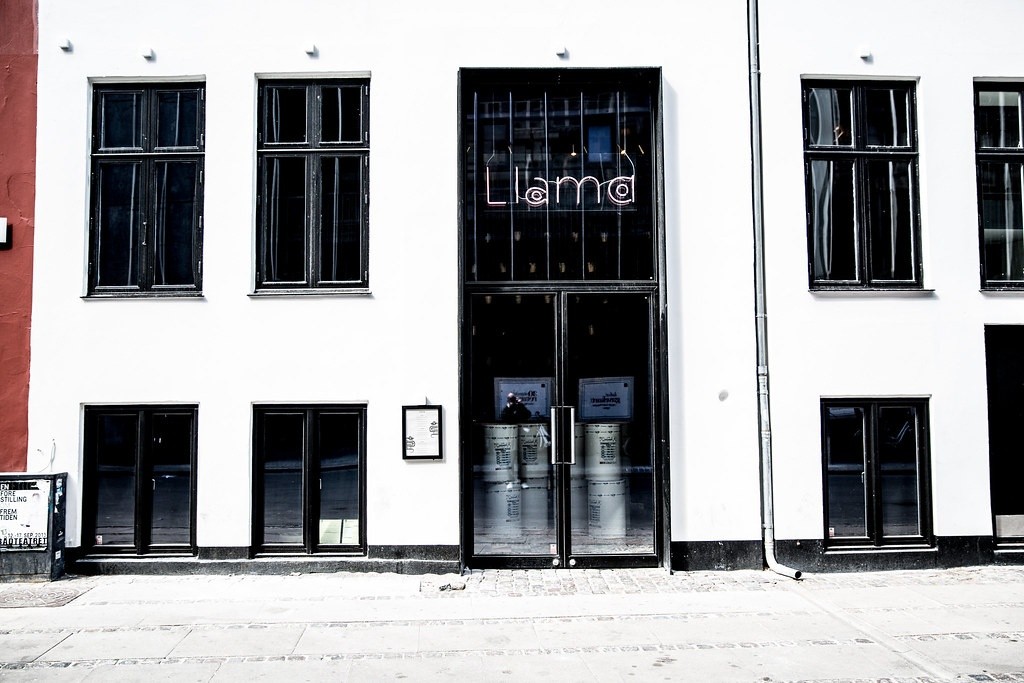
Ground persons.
[499,393,532,424]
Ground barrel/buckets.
[519,423,550,480]
[570,422,586,480]
[482,424,519,481]
[479,478,628,541]
[585,424,621,480]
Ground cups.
[96,535,102,545]
